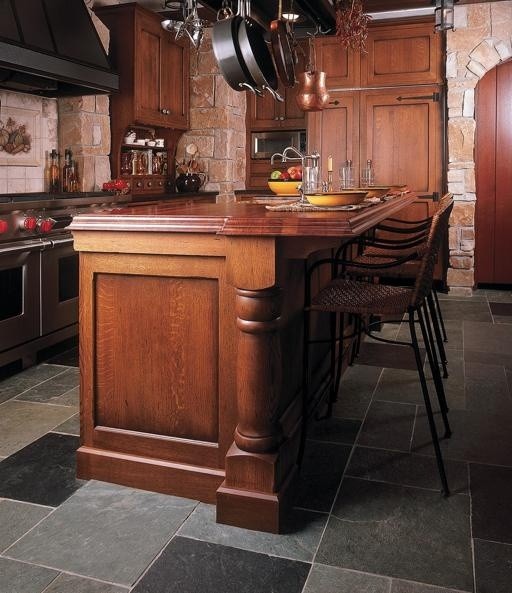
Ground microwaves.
[249,129,307,161]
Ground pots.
[209,0,298,103]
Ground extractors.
[0,0,121,101]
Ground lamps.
[435,0,456,33]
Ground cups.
[339,165,357,189]
[302,165,320,195]
[361,165,377,186]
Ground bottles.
[67,159,80,192]
[63,148,74,191]
[175,170,207,193]
[47,148,60,192]
[294,70,330,111]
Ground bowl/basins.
[267,178,305,195]
[303,184,405,206]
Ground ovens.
[1,214,80,368]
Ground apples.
[271,166,302,180]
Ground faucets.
[269,150,320,189]
[281,146,308,204]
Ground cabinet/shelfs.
[105,3,190,197]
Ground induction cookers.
[0,190,113,211]
